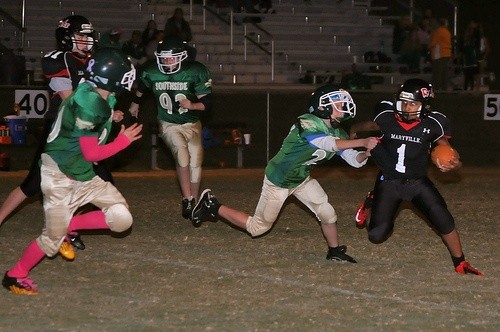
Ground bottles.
[15,104,20,116]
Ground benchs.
[0,0,474,86]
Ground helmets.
[398,79,434,118]
[56,15,97,52]
[309,84,344,118]
[85,47,130,93]
[156,38,185,64]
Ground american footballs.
[431,145,458,167]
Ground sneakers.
[59,241,75,260]
[69,234,85,250]
[455,261,484,276]
[182,198,196,219]
[356,191,375,225]
[192,189,218,228]
[326,246,357,264]
[2,271,38,296]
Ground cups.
[244,134,251,144]
[232,130,241,140]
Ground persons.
[104,8,193,80]
[424,19,452,90]
[191,84,381,263]
[349,78,483,275]
[1,47,143,296]
[129,38,213,220]
[453,35,478,90]
[0,16,124,225]
